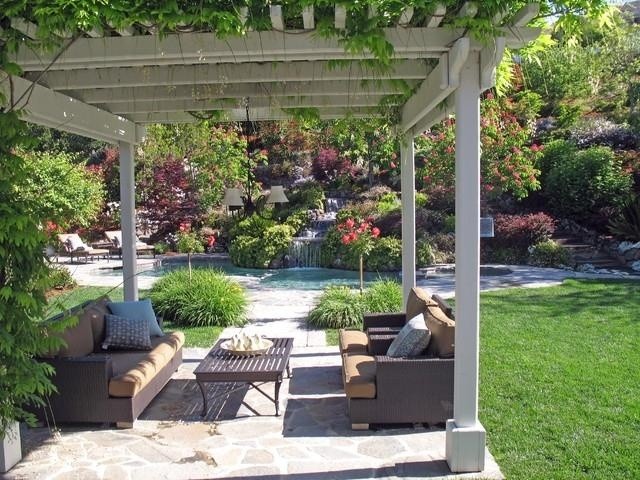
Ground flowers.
[335,217,381,255]
[179,221,216,253]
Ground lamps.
[222,97,290,223]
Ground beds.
[101,298,163,351]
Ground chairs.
[339,285,456,431]
[54,230,157,265]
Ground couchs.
[19,296,185,433]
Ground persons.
[205,235,215,253]
